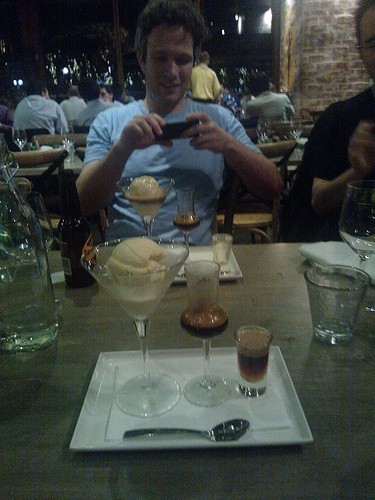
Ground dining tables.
[0,239,375,500]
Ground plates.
[171,246,243,283]
[300,242,374,286]
[70,346,314,449]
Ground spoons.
[125,418,249,440]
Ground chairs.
[5,126,48,152]
[9,144,72,218]
[216,140,299,244]
[240,116,259,143]
[32,132,88,154]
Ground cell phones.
[152,119,200,141]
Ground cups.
[213,232,234,276]
[303,264,369,345]
[235,324,273,396]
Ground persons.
[73,0,283,245]
[243,72,296,143]
[74,78,116,134]
[100,84,114,103]
[293,0,375,241]
[267,79,279,93]
[61,87,89,122]
[186,51,220,104]
[12,80,76,132]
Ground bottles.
[60,169,97,286]
[0,132,61,354]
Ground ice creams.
[126,175,166,216]
[105,237,168,302]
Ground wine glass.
[11,125,27,152]
[339,182,375,273]
[180,260,234,406]
[174,187,200,247]
[289,115,302,151]
[121,174,173,235]
[82,235,190,417]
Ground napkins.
[296,240,375,288]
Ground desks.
[10,154,82,208]
[266,145,308,174]
